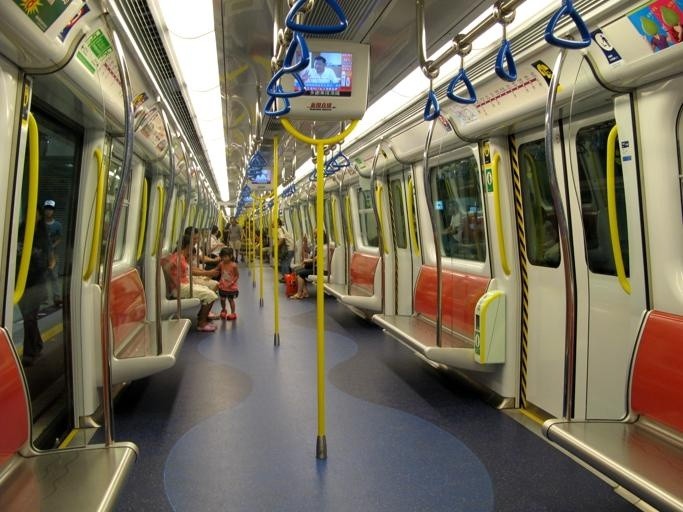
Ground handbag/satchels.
[271,241,288,259]
[285,270,298,297]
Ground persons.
[533,205,556,261]
[168,235,218,333]
[199,216,331,300]
[184,226,219,321]
[444,201,463,259]
[585,203,627,274]
[209,246,239,318]
[298,56,339,85]
[39,198,63,310]
[15,202,49,368]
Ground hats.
[42,199,55,210]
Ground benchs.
[372,264,507,377]
[540,310,683,512]
[323,253,383,311]
[159,263,200,314]
[313,248,344,282]
[0,326,137,511]
[92,268,190,386]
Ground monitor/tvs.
[279,36,371,119]
[246,167,274,192]
[467,206,480,230]
[433,200,446,210]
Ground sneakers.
[21,355,33,366]
[195,309,227,332]
[290,289,309,299]
[226,313,236,319]
